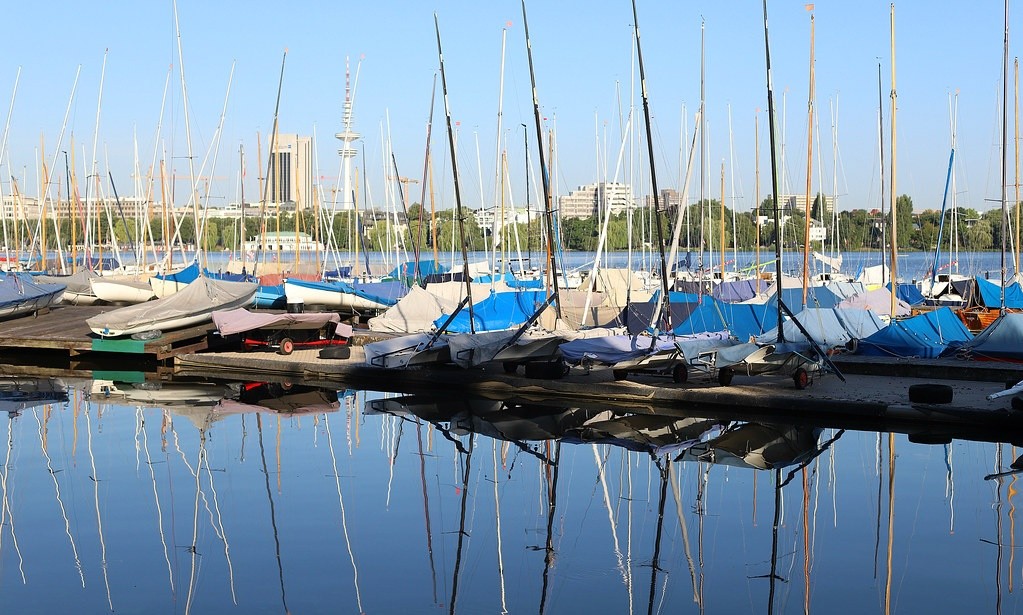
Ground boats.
[0,377,366,615]
[364,0,1023,388]
[364,382,902,615]
[1,0,411,363]
